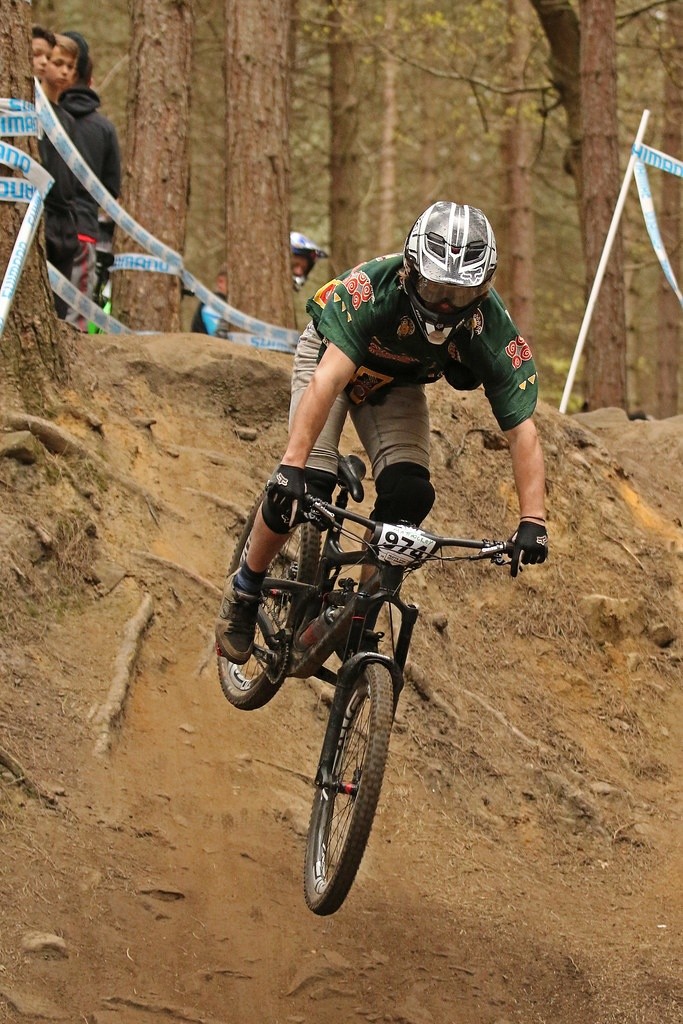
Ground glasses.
[415,272,491,309]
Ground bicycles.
[214,452,549,915]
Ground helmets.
[401,201,499,346]
[289,231,329,293]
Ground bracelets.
[520,516,545,522]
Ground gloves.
[505,517,548,577]
[267,463,307,527]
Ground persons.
[191,231,329,338]
[33,24,123,332]
[215,199,549,665]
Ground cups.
[297,605,344,648]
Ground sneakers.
[361,629,385,654]
[215,567,263,665]
